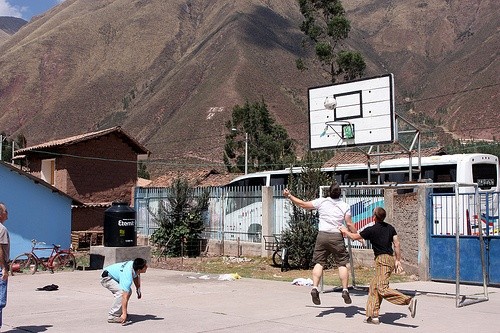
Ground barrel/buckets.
[12,263,20,272]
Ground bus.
[204,153,500,241]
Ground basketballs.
[324,97,338,109]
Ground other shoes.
[342,290,352,304]
[363,317,379,323]
[108,317,121,323]
[311,288,321,305]
[408,298,417,318]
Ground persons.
[101,257,148,324]
[339,206,417,324]
[282,183,364,305]
[0,203,11,327]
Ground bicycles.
[10,238,77,276]
[273,238,290,272]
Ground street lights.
[231,128,248,174]
